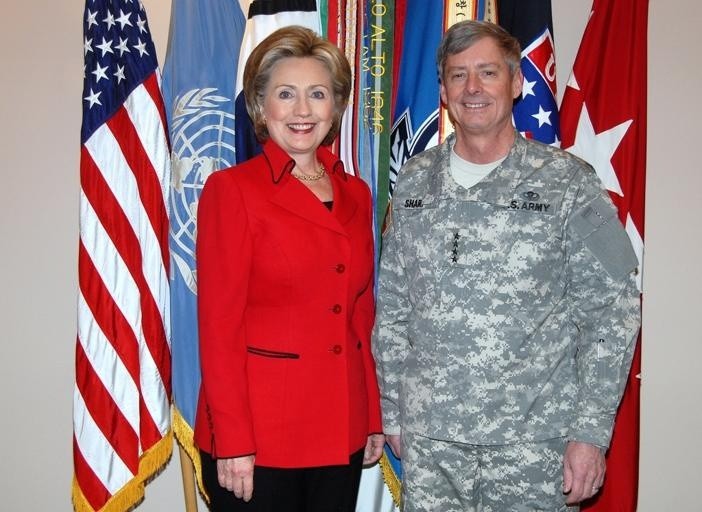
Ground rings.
[592,485,599,490]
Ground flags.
[560,0,648,512]
[157,0,246,504]
[315,0,397,512]
[378,0,497,503]
[73,0,170,511]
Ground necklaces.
[286,169,331,181]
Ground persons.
[499,1,559,147]
[369,21,641,512]
[189,26,383,512]
[233,0,321,167]
